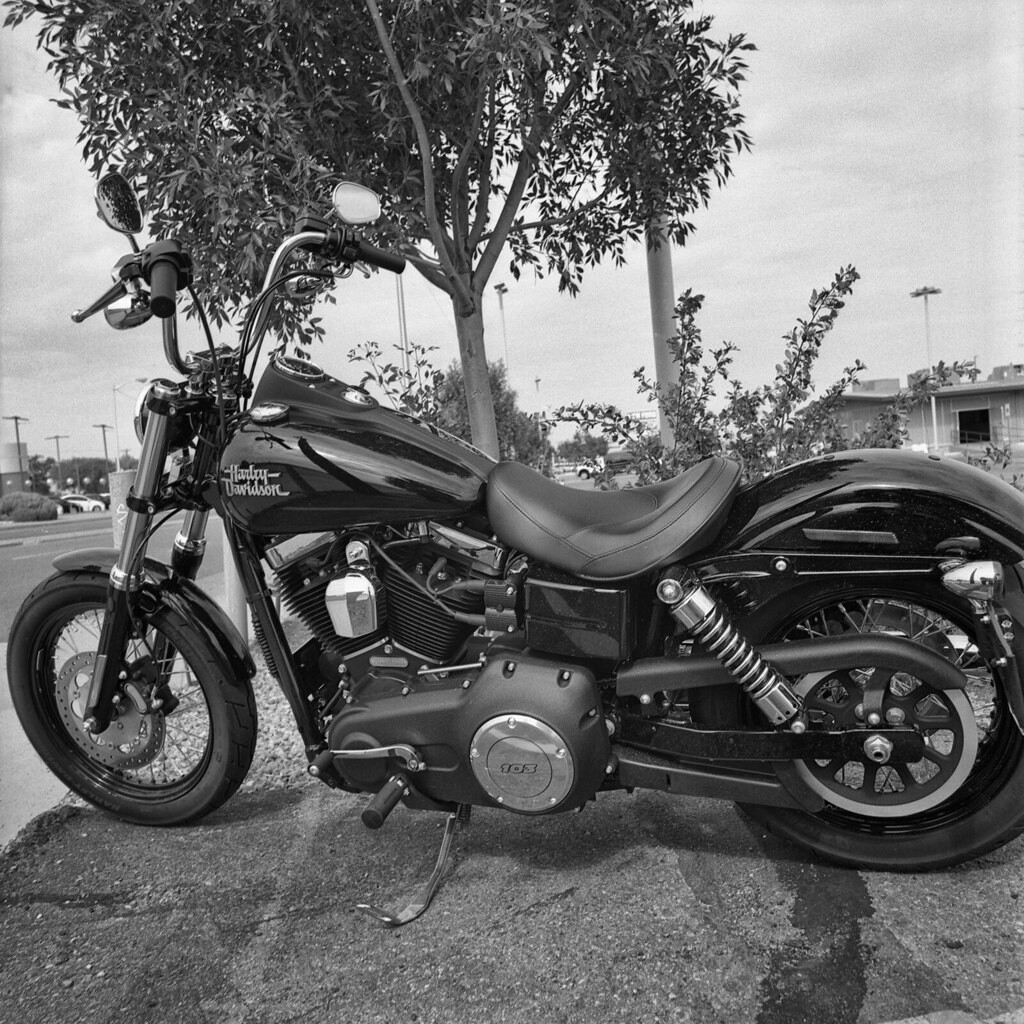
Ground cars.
[574,457,605,482]
[50,492,110,515]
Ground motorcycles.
[5,164,1024,930]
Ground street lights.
[91,425,113,490]
[911,283,944,462]
[493,283,509,391]
[111,375,149,471]
[44,435,70,494]
[2,413,29,494]
